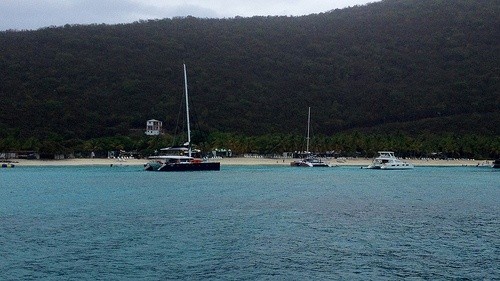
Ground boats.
[0,163,15,167]
[290,106,332,168]
[361,151,414,170]
[143,62,221,172]
